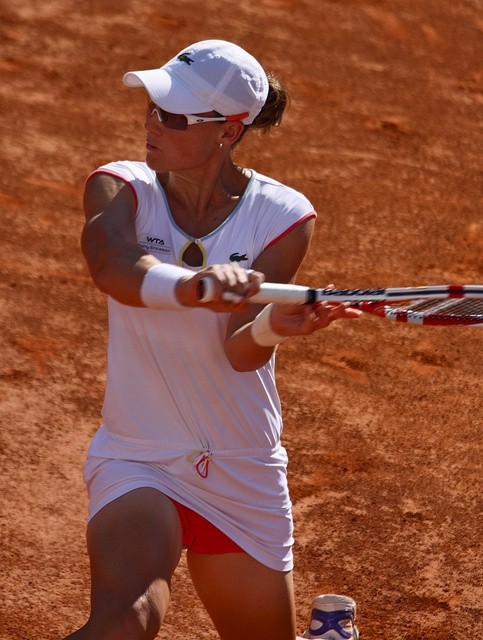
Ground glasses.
[147,96,249,131]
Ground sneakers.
[307,593,358,638]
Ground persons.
[62,37,362,639]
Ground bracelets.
[140,263,196,310]
[251,302,288,347]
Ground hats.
[123,39,268,129]
